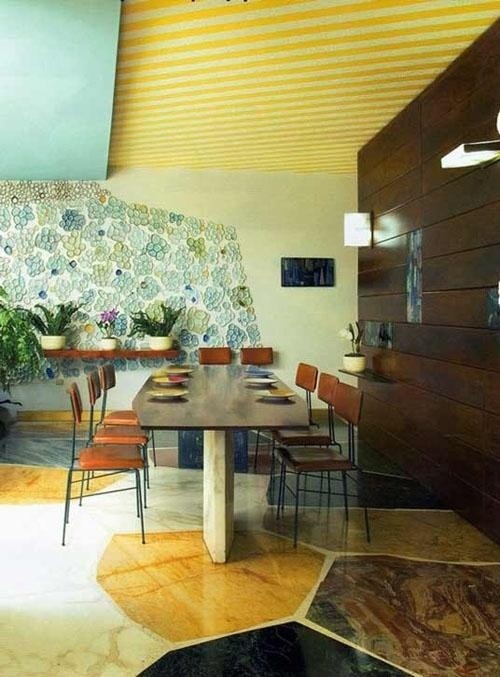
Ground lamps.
[438,137,500,170]
[344,209,374,248]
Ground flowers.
[96,307,123,338]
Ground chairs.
[194,344,275,366]
[61,381,147,545]
[86,364,157,509]
[254,361,341,504]
[276,382,372,549]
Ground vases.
[98,336,118,351]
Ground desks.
[131,365,310,563]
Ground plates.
[144,388,190,399]
[251,387,300,401]
[161,363,195,375]
[151,374,191,389]
[242,367,275,379]
[243,376,280,389]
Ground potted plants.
[126,302,188,352]
[342,319,369,373]
[14,301,86,351]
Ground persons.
[281,256,334,287]
